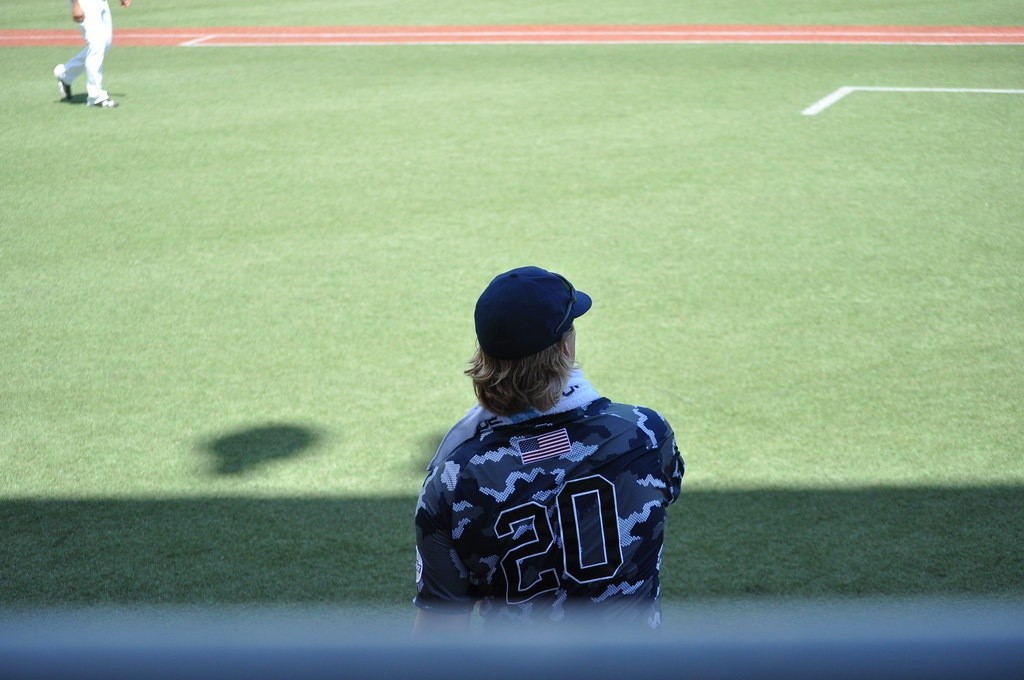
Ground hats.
[475,266,592,360]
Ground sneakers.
[95,99,118,108]
[54,68,72,100]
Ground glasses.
[549,271,577,333]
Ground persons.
[53,0,132,108]
[411,265,685,639]
